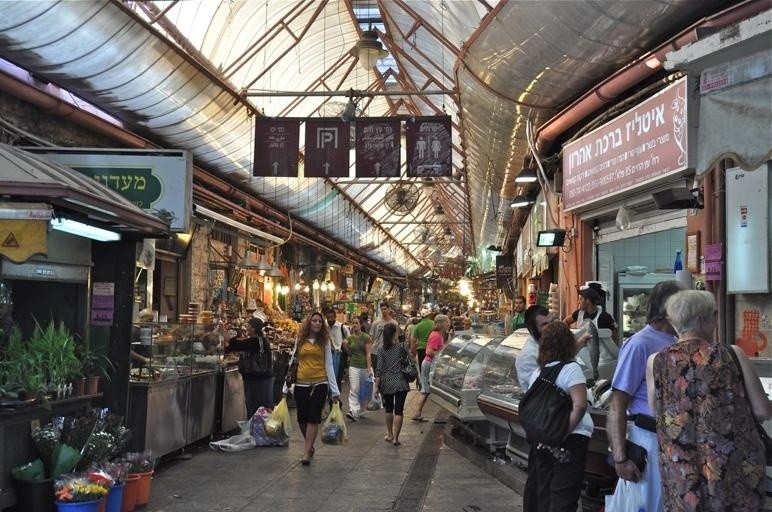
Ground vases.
[12,467,155,512]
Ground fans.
[385,180,418,217]
[414,226,438,245]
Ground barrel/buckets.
[54,471,154,512]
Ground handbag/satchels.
[286,351,299,383]
[399,353,416,382]
[606,439,648,473]
[238,350,274,380]
[519,376,571,443]
[754,423,772,466]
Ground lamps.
[535,228,575,249]
[422,172,454,244]
[487,244,503,252]
[235,246,337,297]
[514,160,538,183]
[509,195,535,208]
[348,0,389,73]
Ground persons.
[644,288,772,512]
[608,276,690,512]
[523,321,595,512]
[563,279,621,334]
[227,292,473,464]
[130,307,157,375]
[514,303,594,512]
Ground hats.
[577,287,603,305]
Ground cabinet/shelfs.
[128,322,191,463]
[478,327,620,474]
[427,332,510,451]
[187,322,223,449]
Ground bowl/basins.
[179,302,212,330]
[140,337,153,345]
[194,355,223,371]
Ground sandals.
[302,409,430,465]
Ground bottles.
[674,249,684,274]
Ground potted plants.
[1,306,122,405]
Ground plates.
[625,266,648,277]
[470,323,483,329]
[654,267,673,274]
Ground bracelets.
[614,458,630,465]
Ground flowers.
[14,407,156,502]
